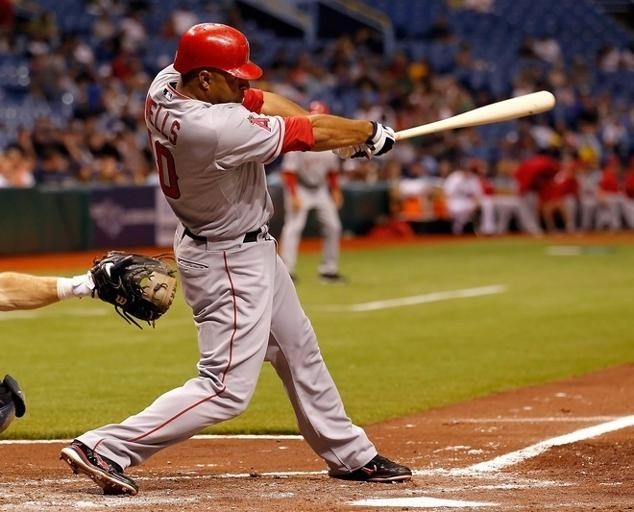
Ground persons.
[221,1,632,240]
[277,98,347,283]
[2,2,199,188]
[0,248,177,322]
[56,20,412,494]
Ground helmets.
[173,22,264,81]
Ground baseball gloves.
[89,250,179,329]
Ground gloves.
[365,121,396,156]
[330,142,373,161]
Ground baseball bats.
[338,90,556,159]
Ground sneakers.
[59,440,138,495]
[328,454,412,482]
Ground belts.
[184,221,269,242]
